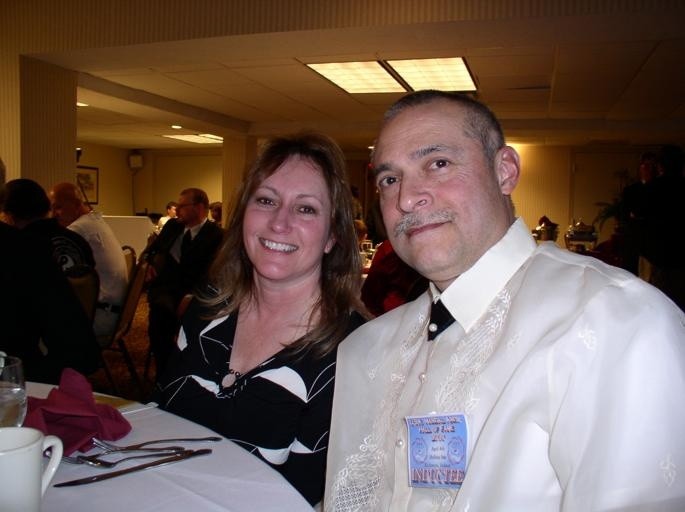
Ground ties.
[180,229,191,256]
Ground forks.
[92,435,222,451]
[61,450,110,464]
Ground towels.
[22,368,134,456]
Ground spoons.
[77,449,193,468]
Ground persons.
[354,220,368,241]
[1,177,130,386]
[637,145,684,312]
[622,151,657,269]
[142,189,226,373]
[153,128,376,506]
[313,89,685,512]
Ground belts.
[97,302,121,314]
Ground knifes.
[52,448,212,488]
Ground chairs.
[97,257,150,402]
[122,246,137,278]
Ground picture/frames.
[76,165,98,205]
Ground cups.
[0,427,64,512]
[0,353,28,426]
